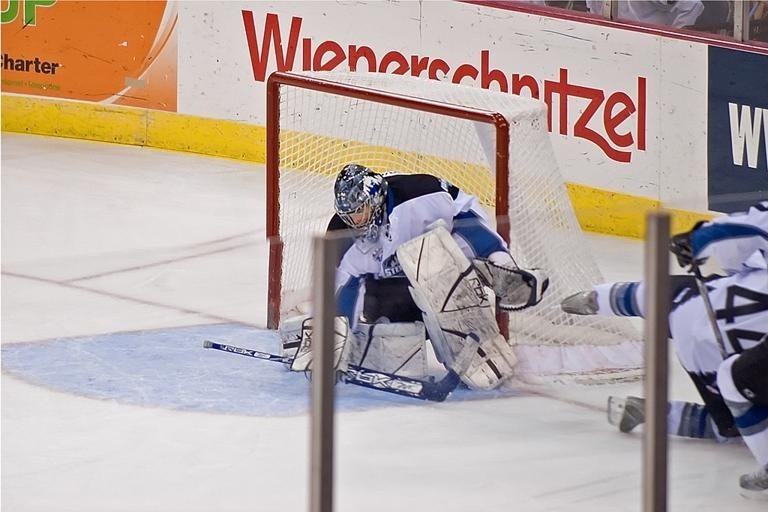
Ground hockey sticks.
[203,332,479,401]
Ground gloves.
[289,314,358,386]
[472,250,549,310]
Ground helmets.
[333,164,389,226]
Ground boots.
[620,394,652,435]
[562,286,602,317]
[739,463,768,491]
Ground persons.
[564,200,768,494]
[518,0,768,43]
[277,162,552,394]
